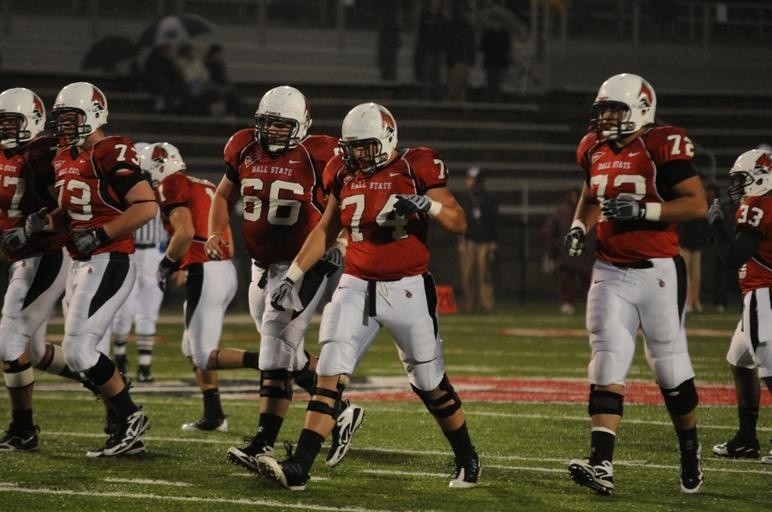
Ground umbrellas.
[132,11,222,62]
[77,33,141,73]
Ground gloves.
[565,226,586,257]
[601,195,646,222]
[707,199,724,227]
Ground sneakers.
[712,433,760,459]
[679,441,704,494]
[449,448,480,488]
[568,459,614,495]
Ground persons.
[537,177,740,320]
[368,1,525,102]
[136,143,266,433]
[105,39,243,122]
[253,101,482,492]
[560,71,711,496]
[204,81,368,473]
[452,163,501,314]
[1,82,178,460]
[704,146,772,464]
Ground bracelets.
[427,200,444,218]
[285,262,303,284]
[208,231,224,237]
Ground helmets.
[0,87,46,151]
[254,86,313,158]
[338,102,398,179]
[590,72,657,144]
[726,149,772,207]
[48,82,108,146]
[141,142,187,186]
